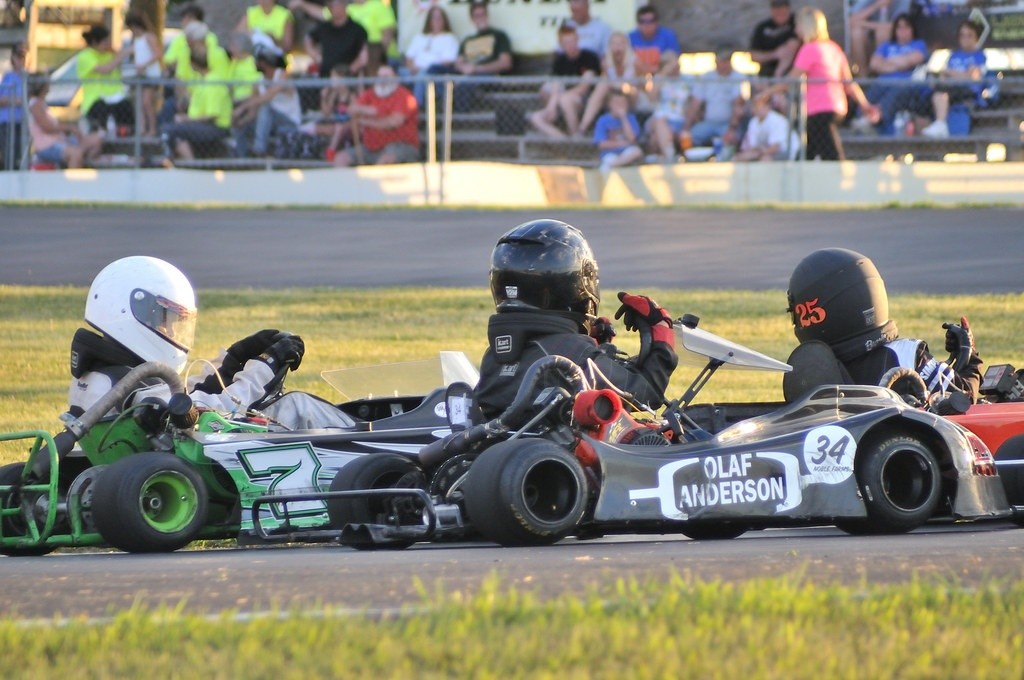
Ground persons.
[527,0,880,173]
[848,0,1000,138]
[787,246,984,405]
[68,255,365,433]
[0,0,514,166]
[473,218,715,445]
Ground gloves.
[942,318,977,355]
[257,334,305,372]
[226,329,290,361]
[590,318,617,342]
[612,291,674,333]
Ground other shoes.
[922,122,949,137]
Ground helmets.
[488,219,601,316]
[785,248,889,345]
[84,257,196,373]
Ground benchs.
[103,75,1021,159]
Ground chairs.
[783,340,854,403]
[445,382,486,435]
[59,408,150,466]
